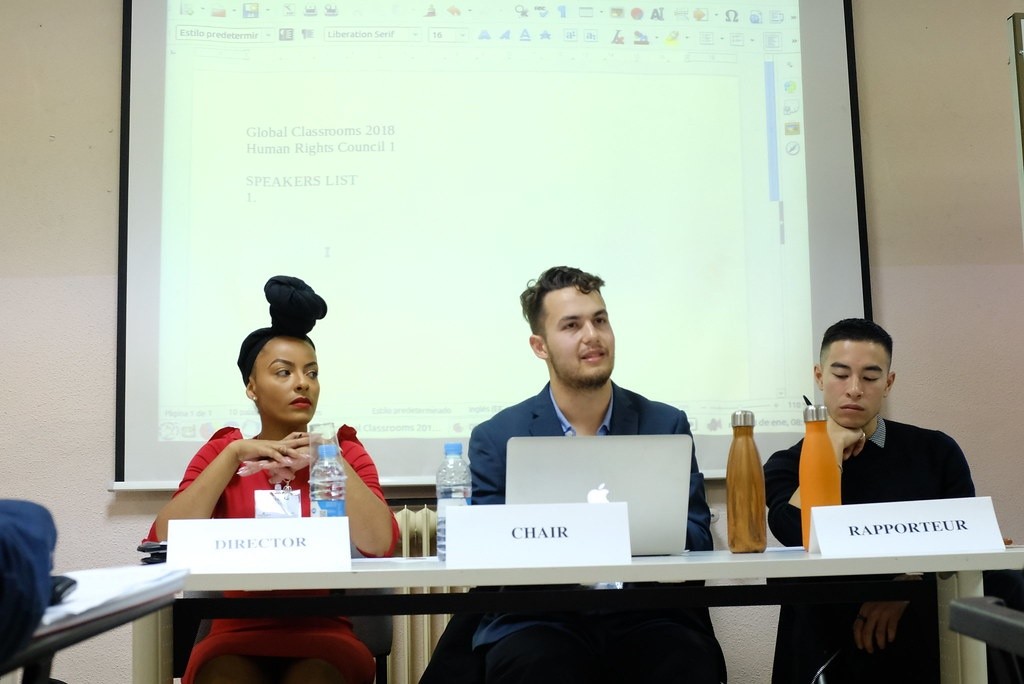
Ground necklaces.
[282,478,292,490]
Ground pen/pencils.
[801,393,812,407]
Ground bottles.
[309,444,346,516]
[798,404,842,552]
[725,411,767,553]
[435,441,473,561]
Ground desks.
[0,544,1024,684]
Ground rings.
[277,444,287,454]
[858,612,867,623]
[860,433,866,441]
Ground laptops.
[504,434,693,554]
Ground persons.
[468,266,714,683]
[142,275,400,684]
[763,318,976,684]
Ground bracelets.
[838,463,843,472]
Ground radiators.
[133,505,480,684]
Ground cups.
[307,422,348,483]
